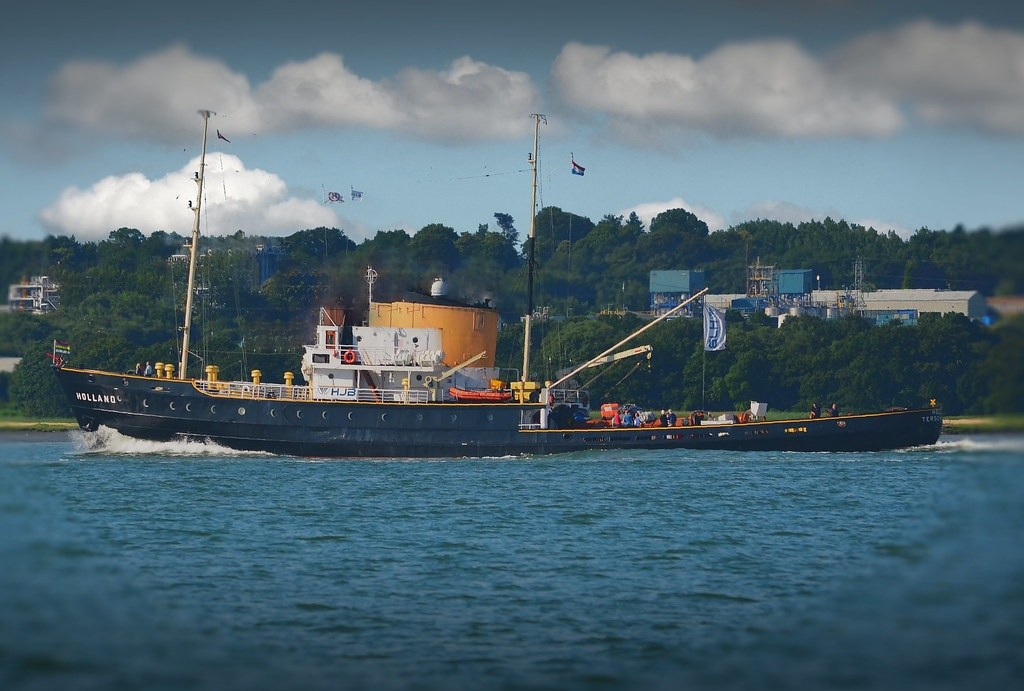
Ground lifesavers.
[344,351,356,363]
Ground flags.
[350,190,364,201]
[329,191,345,203]
[570,160,586,177]
[702,302,728,351]
[216,129,231,143]
[55,340,71,355]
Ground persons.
[696,411,704,422]
[59,357,65,367]
[659,410,668,427]
[830,404,839,417]
[144,361,153,377]
[623,410,633,429]
[614,410,621,428]
[135,363,144,376]
[633,413,640,428]
[810,403,820,419]
[668,409,677,427]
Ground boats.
[50,106,943,461]
